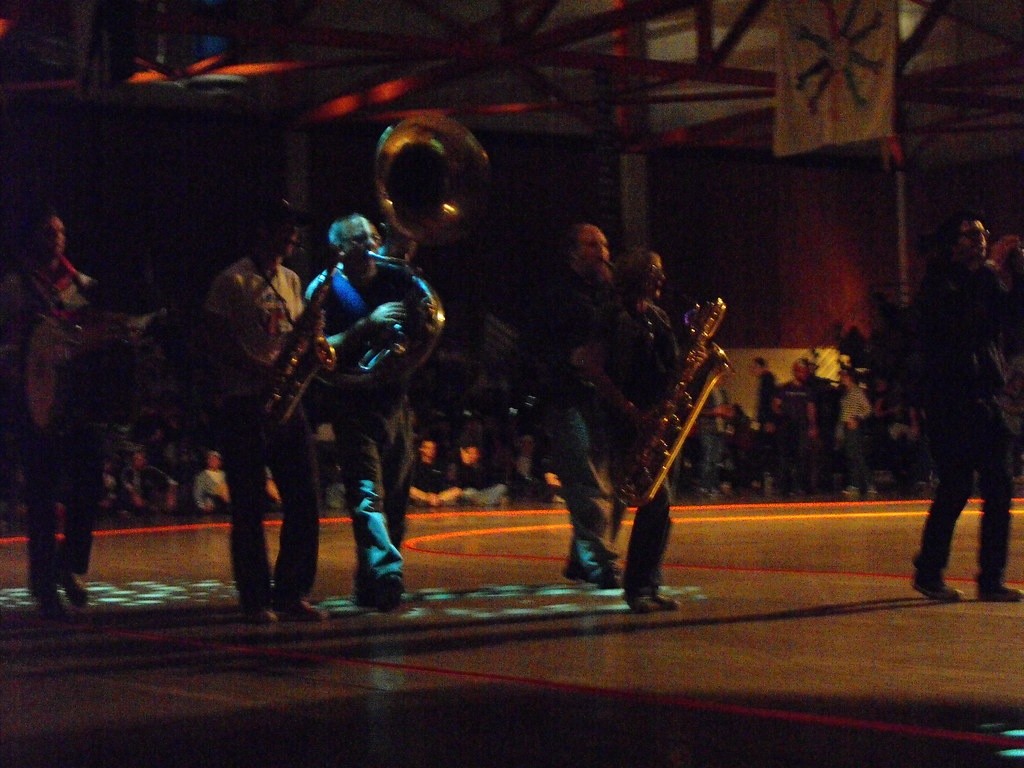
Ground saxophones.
[617,296,731,506]
[261,252,342,430]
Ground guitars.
[25,305,167,430]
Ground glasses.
[958,229,991,241]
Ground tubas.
[337,110,494,390]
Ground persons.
[0,195,1024,624]
[912,209,1024,603]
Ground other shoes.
[562,560,625,588]
[352,579,377,607]
[46,558,89,607]
[37,592,69,622]
[653,592,681,612]
[242,602,280,624]
[624,594,662,613]
[376,573,405,611]
[911,570,964,601]
[276,598,330,622]
[973,586,1024,603]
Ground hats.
[252,196,311,225]
